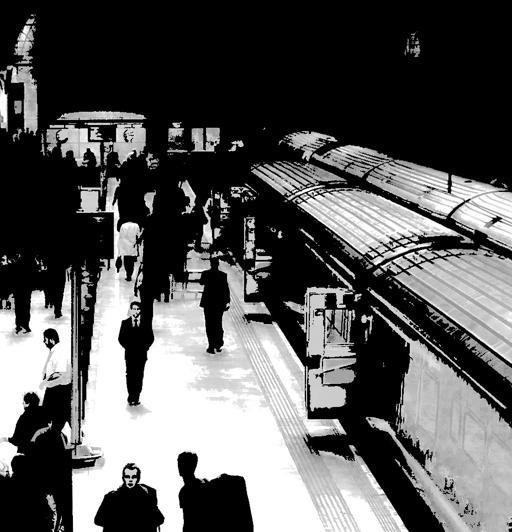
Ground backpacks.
[185,475,254,532]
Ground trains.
[247,158,511,424]
[276,129,511,253]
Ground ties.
[134,319,138,329]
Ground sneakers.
[0,303,64,334]
[123,275,132,282]
[207,348,222,354]
[155,295,170,302]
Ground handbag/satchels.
[115,256,122,273]
